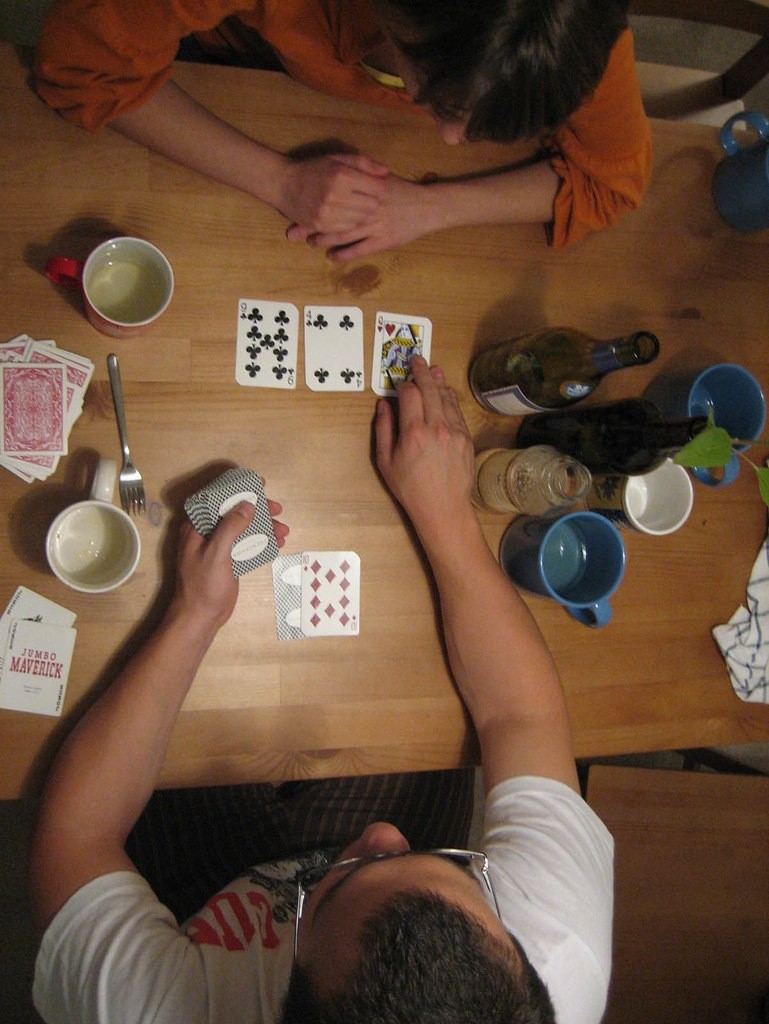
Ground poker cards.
[235,297,298,390]
[0,584,79,716]
[0,332,95,486]
[303,304,365,392]
[370,311,433,397]
[183,466,280,579]
[271,550,361,642]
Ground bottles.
[470,326,660,417]
[468,444,593,514]
[514,398,708,476]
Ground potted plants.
[644,364,769,505]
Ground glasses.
[293,848,503,957]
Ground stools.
[586,766,769,1024]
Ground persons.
[32,0,653,259]
[30,356,617,1024]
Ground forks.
[107,353,147,516]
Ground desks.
[0,43,769,801]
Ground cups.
[45,459,141,593]
[82,237,174,339]
[712,111,769,232]
[500,509,624,627]
[583,457,693,536]
[643,363,765,488]
